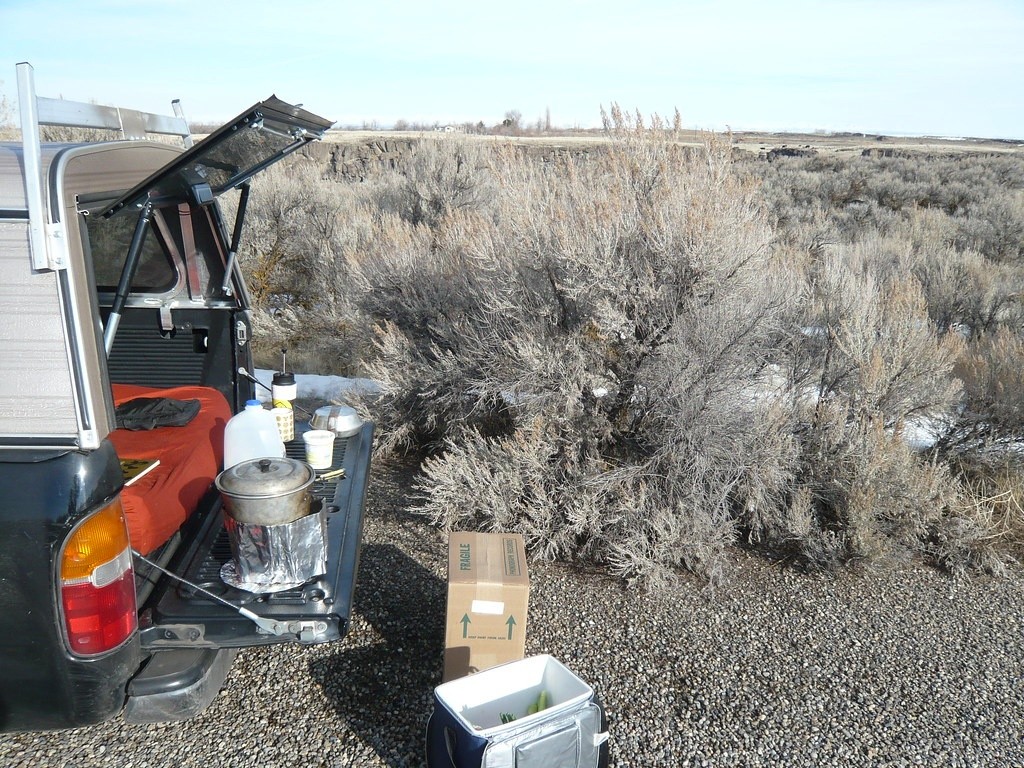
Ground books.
[119,459,160,486]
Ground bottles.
[224,399,286,470]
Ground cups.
[302,429,336,470]
[272,373,297,413]
[270,408,294,443]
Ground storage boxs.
[443,532,529,683]
[434,654,601,768]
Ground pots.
[214,456,345,527]
[308,404,363,438]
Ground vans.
[0,60,377,733]
